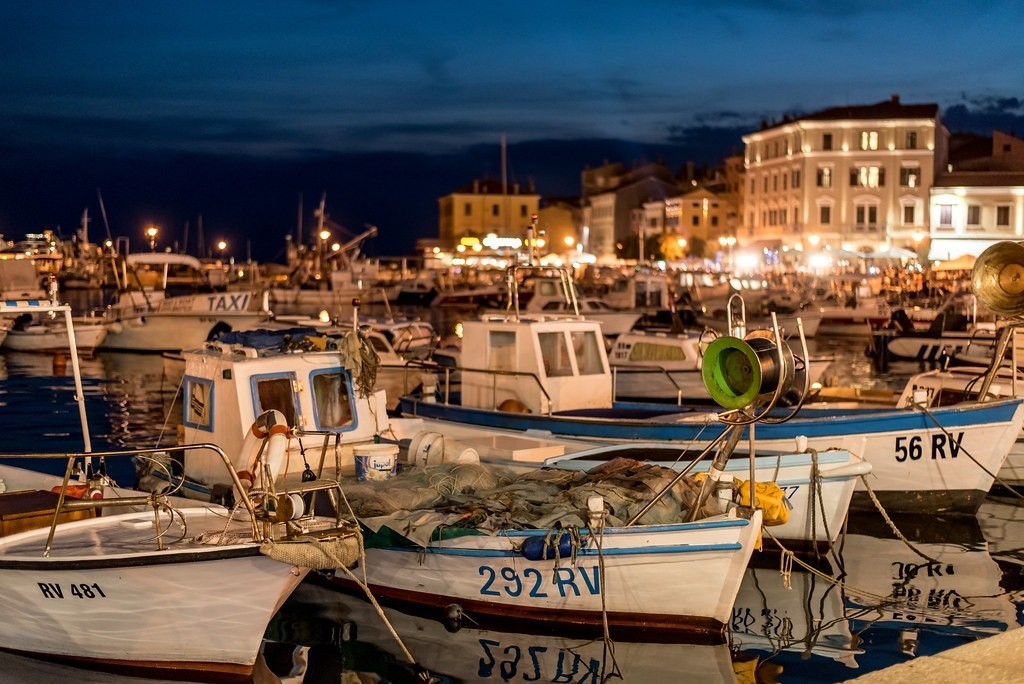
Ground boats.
[267,573,741,684]
[0,205,1024,552]
[720,549,865,669]
[827,510,1020,642]
[132,336,760,646]
[1,297,364,679]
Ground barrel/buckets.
[353,444,399,482]
[407,430,480,466]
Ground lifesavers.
[50,483,103,502]
[230,408,289,509]
